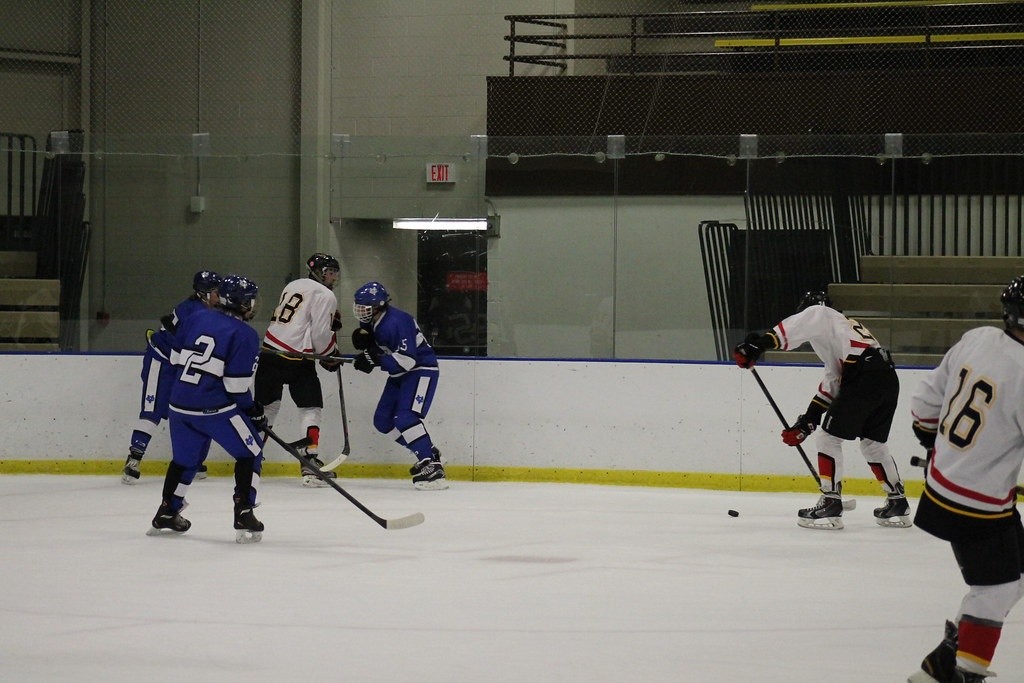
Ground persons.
[253,252,344,488]
[733,289,913,530]
[121,270,222,486]
[146,275,268,544]
[352,282,449,490]
[908,274,1024,683]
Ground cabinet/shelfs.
[0,249,62,355]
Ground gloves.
[780,414,817,447]
[249,402,268,431]
[319,349,345,373]
[733,333,766,368]
[353,350,380,374]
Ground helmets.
[352,282,389,323]
[219,275,259,320]
[192,271,222,305]
[1001,274,1024,326]
[796,290,828,312]
[305,253,339,289]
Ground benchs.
[763,256,1024,369]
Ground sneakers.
[145,499,191,536]
[232,491,265,543]
[411,458,450,489]
[195,466,207,479]
[907,619,998,682]
[797,481,845,530]
[298,452,337,487]
[874,481,913,528]
[120,453,142,485]
[410,445,448,475]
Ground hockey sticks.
[319,329,351,472]
[144,328,356,361]
[263,424,425,530]
[740,346,857,511]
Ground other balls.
[728,510,739,517]
